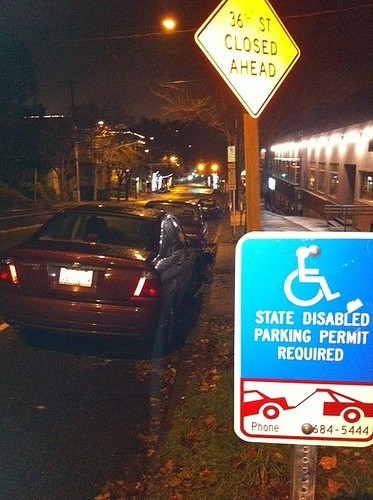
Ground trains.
[260,117,373,233]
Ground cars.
[143,200,212,262]
[179,188,229,221]
[0,201,207,357]
